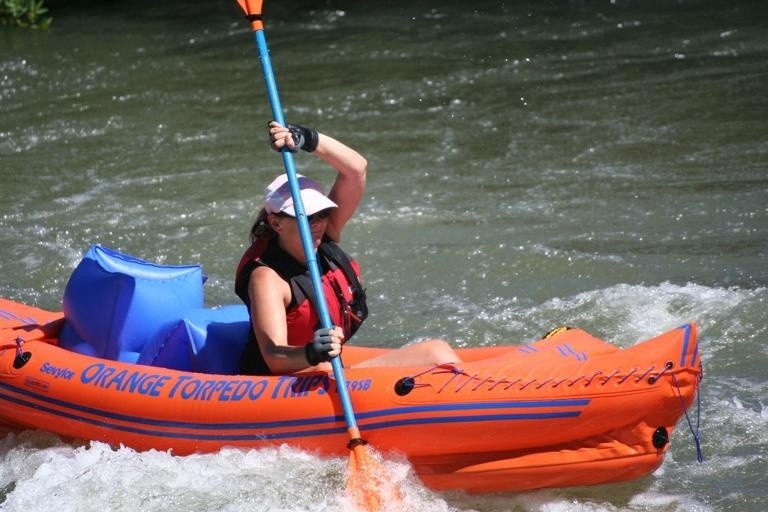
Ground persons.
[233,120,465,377]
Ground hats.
[261,172,339,219]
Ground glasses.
[276,210,332,219]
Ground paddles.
[240,1,404,512]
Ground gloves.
[305,327,340,366]
[267,118,318,154]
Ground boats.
[1,295,702,491]
